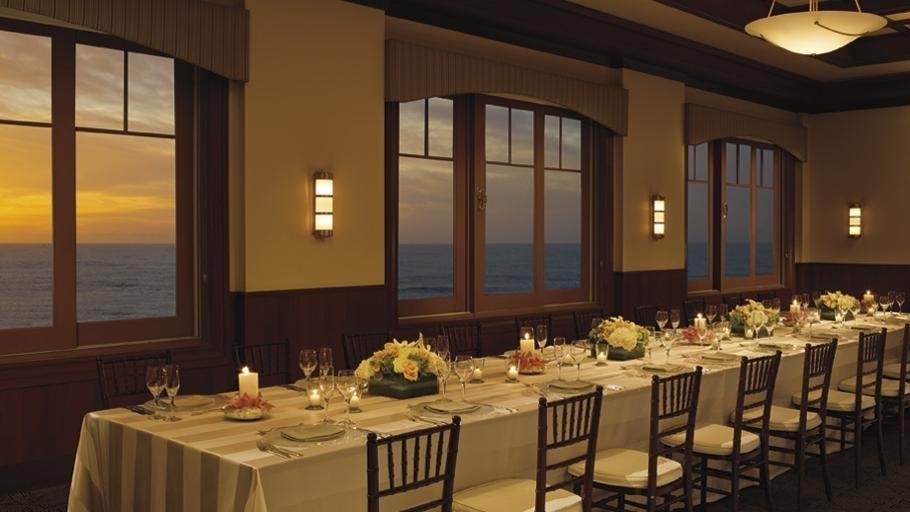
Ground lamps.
[847,200,864,239]
[648,191,667,241]
[312,170,334,240]
[744,1,891,59]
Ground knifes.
[404,411,448,426]
[124,403,153,416]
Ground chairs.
[361,412,463,512]
[73,288,896,409]
[656,348,785,509]
[791,324,893,484]
[725,337,842,497]
[563,364,705,508]
[446,383,606,511]
[837,318,909,449]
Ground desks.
[219,293,909,504]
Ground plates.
[145,396,213,407]
[279,426,346,442]
[547,380,593,389]
[424,400,480,413]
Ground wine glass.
[422,332,485,401]
[299,347,364,426]
[504,290,907,383]
[146,364,181,422]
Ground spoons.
[255,438,303,459]
